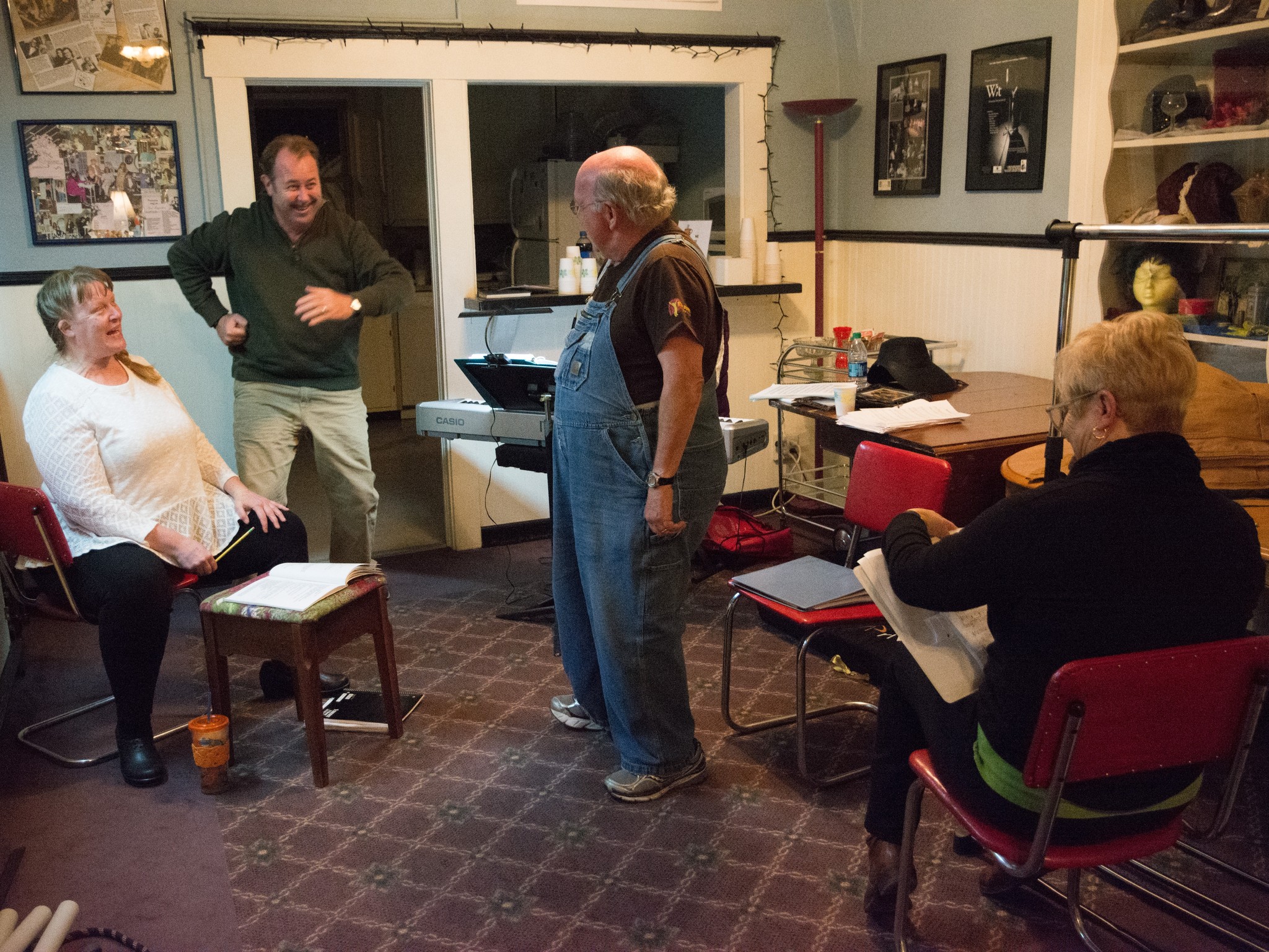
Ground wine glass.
[1160,93,1188,133]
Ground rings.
[321,305,326,314]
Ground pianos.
[414,395,771,468]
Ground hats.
[868,337,959,395]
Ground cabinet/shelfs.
[1095,1,1268,354]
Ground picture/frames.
[6,0,178,96]
[874,52,949,196]
[15,117,187,249]
[963,36,1055,193]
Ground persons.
[551,146,732,806]
[21,266,350,788]
[863,311,1264,917]
[1131,245,1184,313]
[166,134,415,561]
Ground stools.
[200,560,403,789]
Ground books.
[850,523,998,706]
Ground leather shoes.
[258,662,350,700]
[977,861,1061,894]
[863,836,917,915]
[115,728,168,786]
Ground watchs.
[351,294,362,315]
[644,472,674,488]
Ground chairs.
[887,636,1269,951]
[720,440,949,788]
[0,483,226,767]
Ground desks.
[782,370,1067,531]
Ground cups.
[187,712,231,794]
[739,213,781,285]
[558,244,598,297]
[832,382,857,418]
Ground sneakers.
[602,735,707,802]
[550,692,609,731]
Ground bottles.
[833,326,852,369]
[577,231,592,259]
[848,333,867,386]
[1244,281,1269,338]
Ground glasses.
[569,196,610,214]
[1045,389,1102,433]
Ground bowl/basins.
[794,335,835,357]
